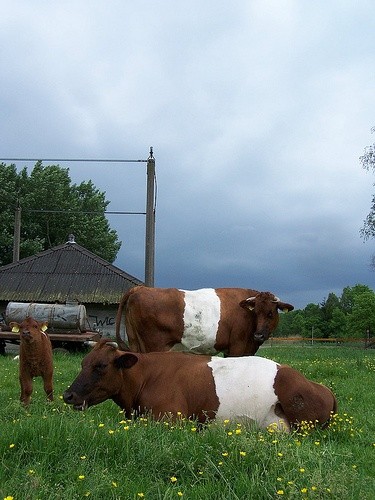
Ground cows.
[9,317,56,409]
[115,285,295,355]
[62,340,338,431]
